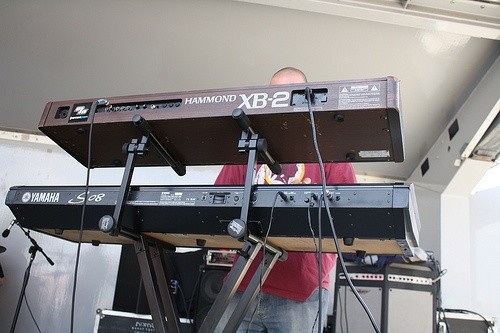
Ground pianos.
[39,75,405,169]
[6,181,421,258]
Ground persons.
[211,64,356,333]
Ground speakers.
[193,266,232,331]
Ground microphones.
[2,218,16,237]
